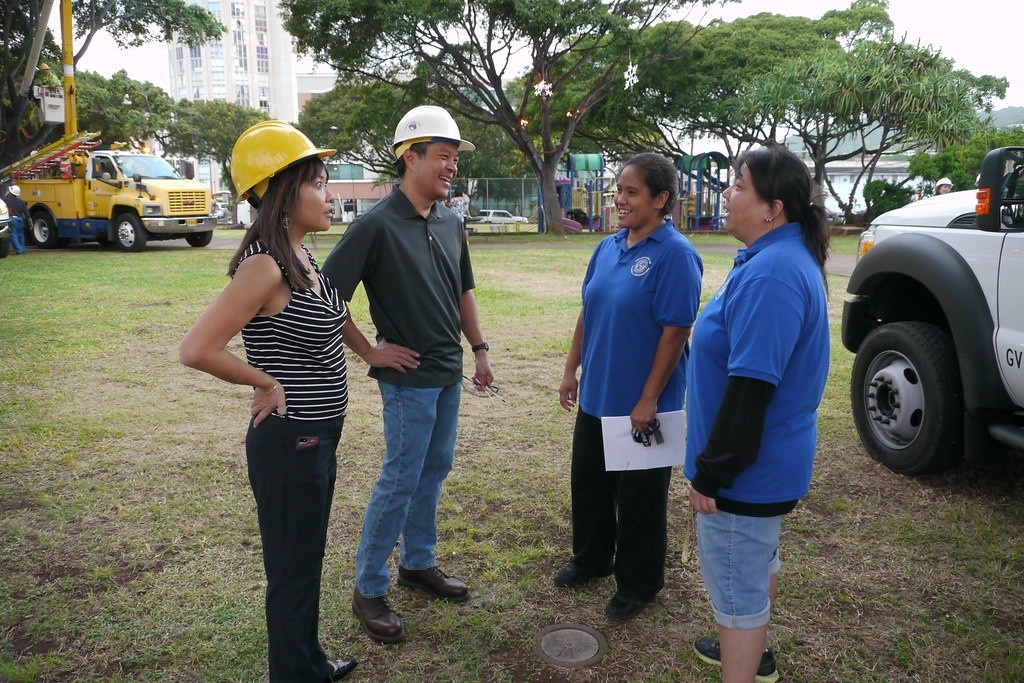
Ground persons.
[5,185,34,255]
[552,154,703,620]
[321,106,494,645]
[180,123,357,683]
[682,145,831,683]
[934,178,954,196]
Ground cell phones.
[295,436,320,450]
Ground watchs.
[472,342,489,352]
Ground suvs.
[0,199,11,260]
[475,209,529,224]
[215,204,232,218]
[840,145,1024,480]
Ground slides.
[562,217,582,233]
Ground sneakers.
[695,636,780,682]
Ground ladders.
[0,130,100,177]
[0,140,102,186]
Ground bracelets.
[256,386,276,395]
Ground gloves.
[27,218,33,230]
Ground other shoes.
[606,582,661,617]
[556,558,616,586]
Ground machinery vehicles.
[0,130,217,251]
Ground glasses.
[461,374,500,398]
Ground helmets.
[936,177,953,186]
[9,185,21,196]
[228,121,336,201]
[391,106,476,156]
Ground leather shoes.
[397,565,468,599]
[329,658,357,679]
[353,588,405,644]
